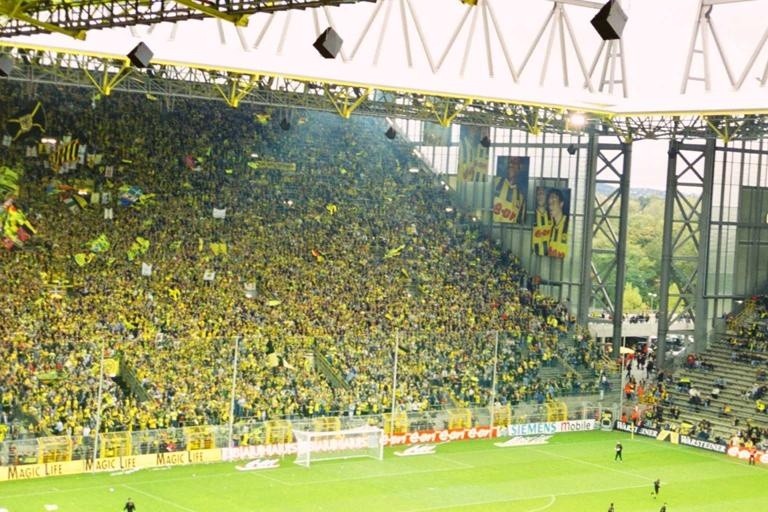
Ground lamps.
[126,40,157,71]
[590,1,629,43]
[312,27,345,61]
